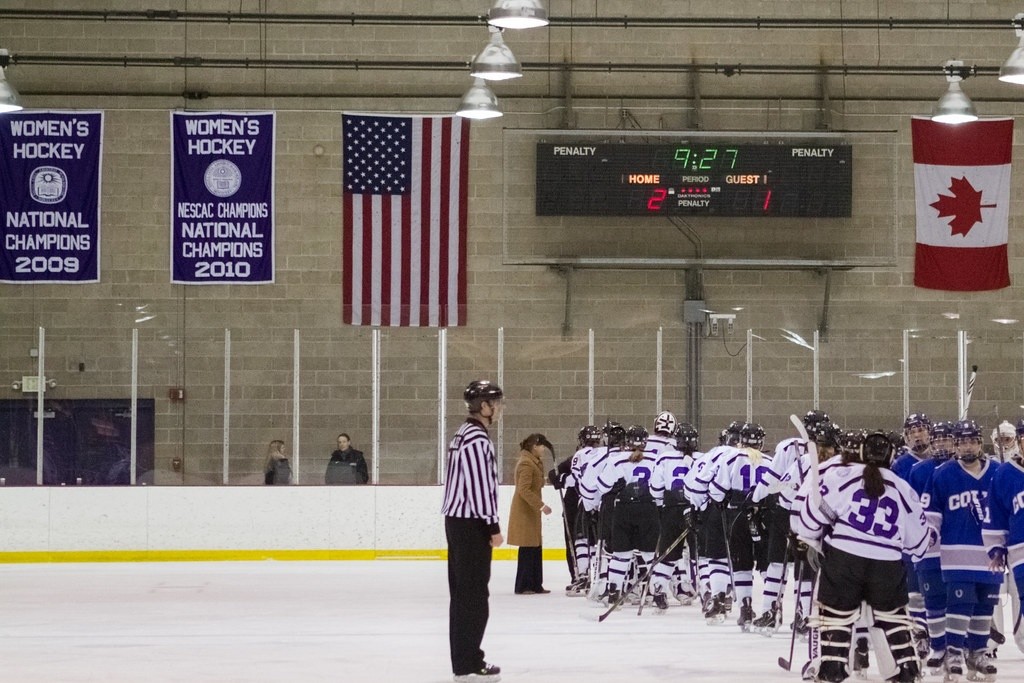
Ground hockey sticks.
[580,365,1004,671]
[537,437,581,580]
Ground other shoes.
[535,587,551,593]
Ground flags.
[910,117,1011,292]
[342,114,470,327]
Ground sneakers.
[909,627,1005,683]
[853,626,868,680]
[452,664,501,683]
[565,575,807,636]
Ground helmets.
[739,423,766,452]
[463,381,503,403]
[929,421,956,443]
[674,422,699,451]
[602,422,626,446]
[625,425,649,448]
[577,425,600,446]
[1016,416,1024,434]
[718,421,746,447]
[653,410,676,436]
[990,420,1019,459]
[952,421,983,443]
[903,413,931,432]
[804,410,905,465]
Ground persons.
[507,434,551,594]
[441,381,504,683]
[325,433,368,484]
[264,440,290,486]
[550,410,1024,683]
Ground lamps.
[11,375,57,392]
[455,76,503,119]
[487,0,550,29]
[999,14,1024,84]
[931,59,978,125]
[470,26,523,81]
[0,48,23,111]
